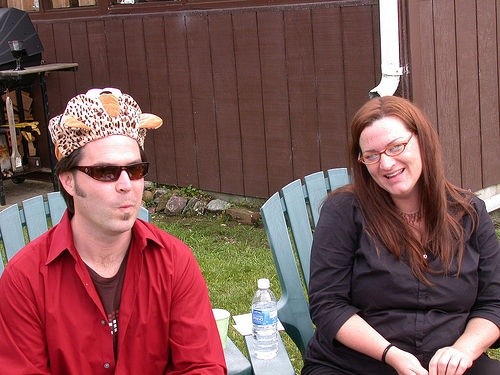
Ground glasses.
[357,131,415,164]
[73,162,150,182]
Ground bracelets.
[382,344,392,363]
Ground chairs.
[0,191,252,375]
[242,168,356,375]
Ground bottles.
[252,278,278,360]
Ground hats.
[47,87,164,160]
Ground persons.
[301,96,500,375]
[0,88,228,375]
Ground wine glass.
[8,40,25,71]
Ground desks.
[1,61,79,203]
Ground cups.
[212,309,230,350]
